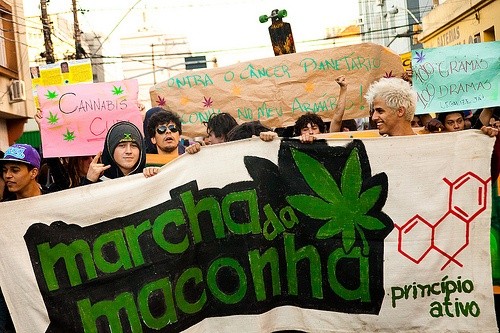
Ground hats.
[0,144,41,168]
[108,124,141,158]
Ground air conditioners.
[6,80,26,103]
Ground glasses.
[76,157,89,160]
[155,125,179,135]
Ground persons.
[30,67,40,79]
[61,62,69,73]
[34,72,500,194]
[0,143,41,202]
